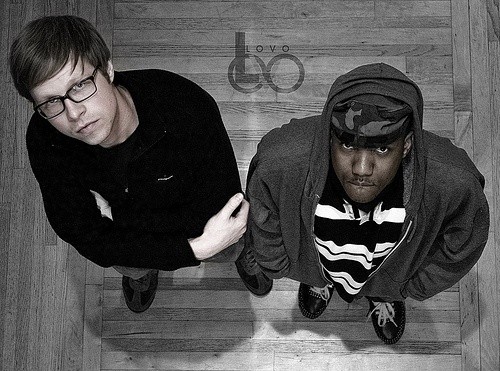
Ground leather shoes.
[298,282,333,319]
[364,295,406,344]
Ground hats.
[329,93,414,147]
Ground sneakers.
[122,268,159,313]
[235,248,273,296]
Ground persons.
[245,63,490,346]
[10,16,250,314]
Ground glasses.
[32,59,102,120]
